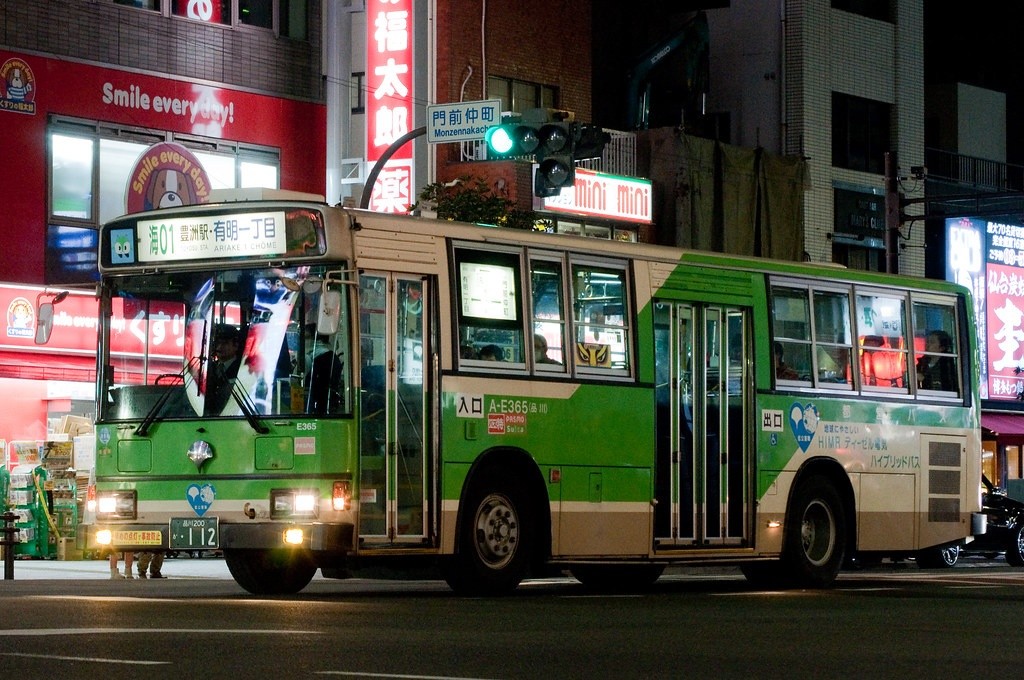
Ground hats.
[214,324,241,343]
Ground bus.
[34,187,986,592]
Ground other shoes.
[140,574,147,579]
[150,573,167,578]
[126,574,134,579]
[112,574,125,580]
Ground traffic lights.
[484,121,577,189]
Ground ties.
[218,363,224,378]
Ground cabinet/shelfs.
[0,438,90,560]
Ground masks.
[535,350,543,362]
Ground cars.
[916,471,1024,569]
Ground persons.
[111,553,134,580]
[774,343,799,381]
[305,323,342,416]
[535,334,562,365]
[137,551,168,579]
[916,330,957,392]
[707,333,743,394]
[204,323,241,417]
[459,329,509,362]
